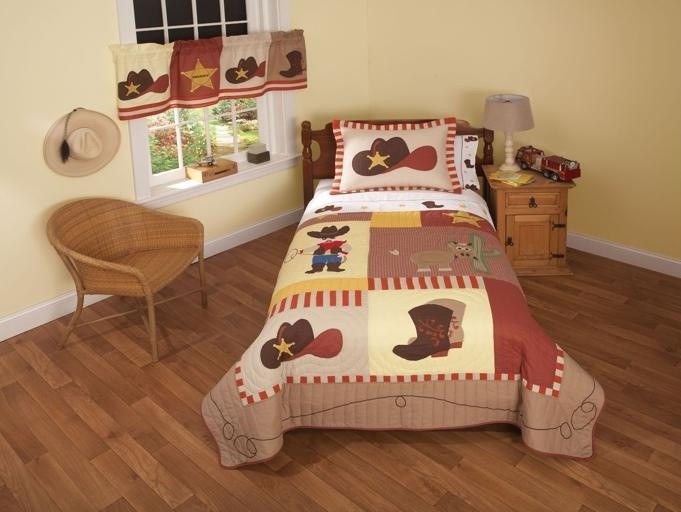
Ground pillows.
[329,117,482,196]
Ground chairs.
[45,196,210,363]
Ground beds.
[201,118,607,469]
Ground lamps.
[483,93,536,173]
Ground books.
[489,169,536,186]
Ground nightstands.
[482,163,577,278]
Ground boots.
[422,200,444,208]
[391,299,465,362]
[279,50,302,78]
[304,263,324,274]
[327,262,345,272]
[464,159,474,168]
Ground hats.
[464,136,477,142]
[351,137,437,176]
[118,68,169,101]
[261,320,343,369]
[43,108,122,177]
[225,56,266,84]
[465,184,477,191]
[314,205,341,213]
[308,225,350,238]
